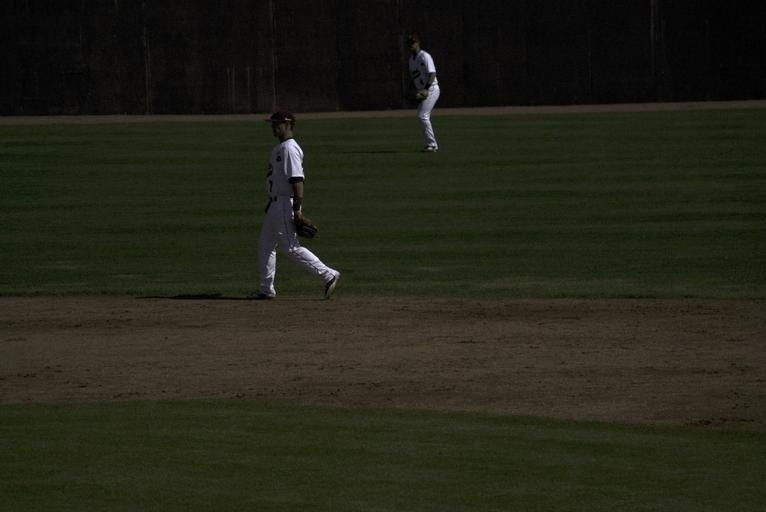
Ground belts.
[270,196,277,202]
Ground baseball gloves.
[414,88,429,100]
[294,214,318,238]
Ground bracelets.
[424,82,431,90]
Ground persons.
[246,109,340,300]
[406,31,442,151]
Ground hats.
[265,111,295,122]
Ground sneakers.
[246,291,277,299]
[325,272,341,298]
[423,147,438,152]
